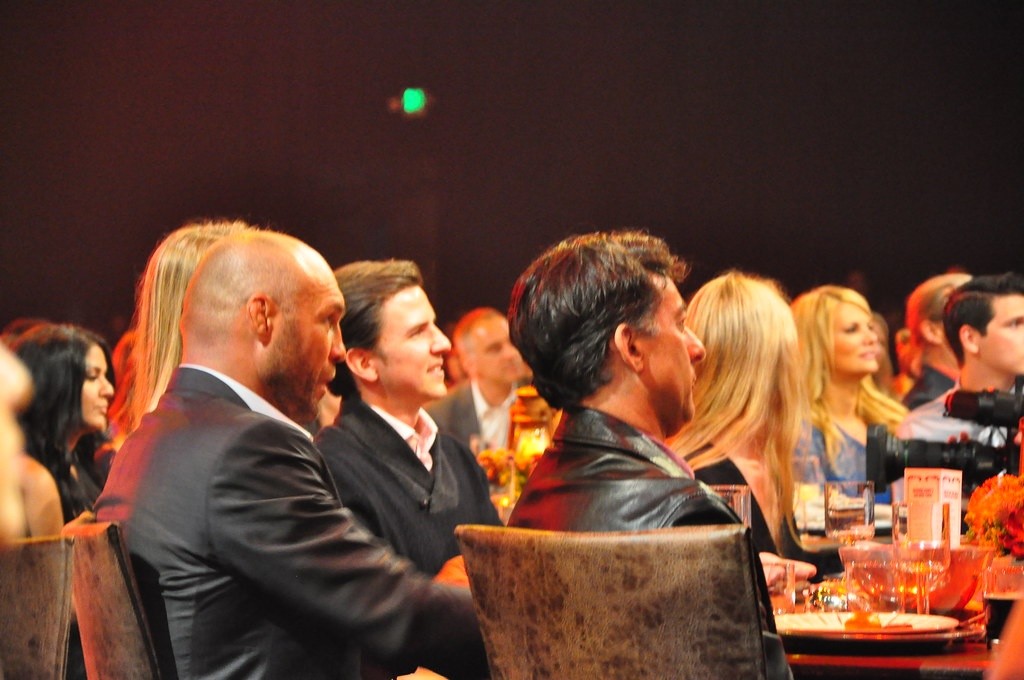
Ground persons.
[0,222,1023,680]
[507,232,795,680]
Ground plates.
[775,611,960,634]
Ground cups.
[761,562,795,616]
[709,484,751,531]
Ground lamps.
[507,385,550,475]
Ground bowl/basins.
[838,541,997,613]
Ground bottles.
[511,416,550,503]
[507,386,551,463]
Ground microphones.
[944,389,1024,428]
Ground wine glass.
[892,502,951,616]
[824,482,875,584]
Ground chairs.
[0,535,75,680]
[453,520,795,680]
[63,510,165,680]
